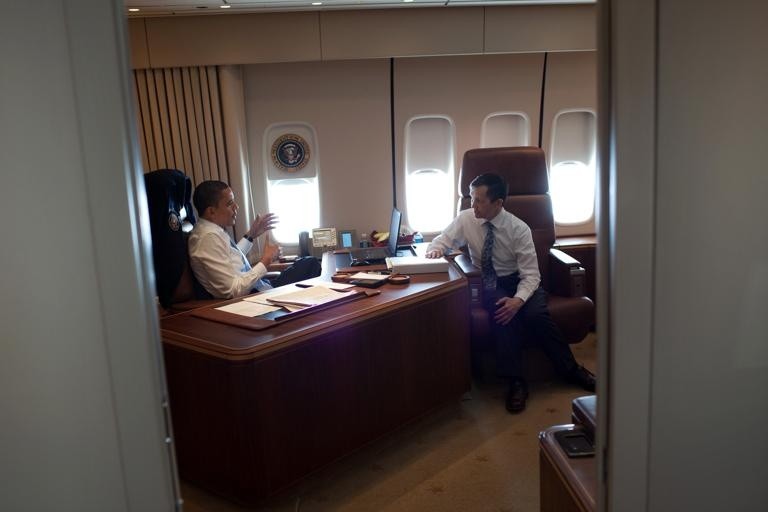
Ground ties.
[224,229,273,292]
[480,221,497,293]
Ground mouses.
[350,261,369,267]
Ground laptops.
[349,206,401,263]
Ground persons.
[423,171,598,414]
[186,179,323,300]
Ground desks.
[159,242,472,512]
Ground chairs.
[144,169,214,308]
[453,146,596,390]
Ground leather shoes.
[505,380,527,413]
[568,365,595,393]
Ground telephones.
[299,227,337,261]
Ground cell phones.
[559,433,596,459]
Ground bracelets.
[243,233,254,243]
